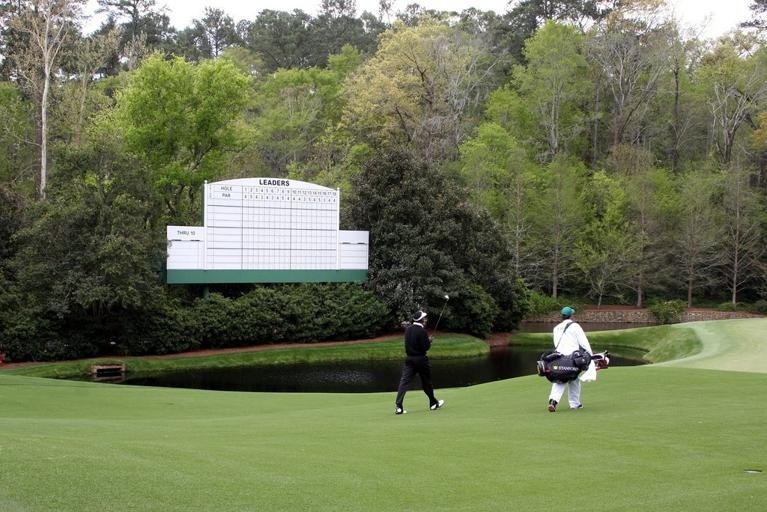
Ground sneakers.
[547,399,557,412]
[570,403,583,409]
[395,407,407,414]
[430,400,445,410]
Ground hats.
[413,310,428,322]
[561,307,575,316]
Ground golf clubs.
[431,295,450,343]
[591,350,611,370]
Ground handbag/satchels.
[577,359,597,383]
[535,349,592,384]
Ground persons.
[395,311,444,414]
[546,306,596,412]
